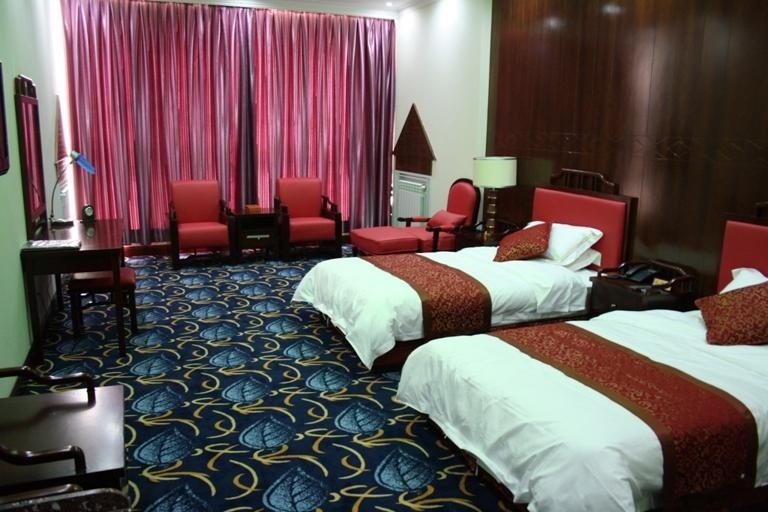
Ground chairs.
[397,178,481,251]
[273,177,342,259]
[0,366,132,512]
[165,179,235,270]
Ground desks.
[227,206,280,257]
[20,219,128,358]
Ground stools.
[67,267,138,334]
[350,227,418,257]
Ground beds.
[391,216,768,512]
[290,167,639,372]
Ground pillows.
[719,267,768,294]
[494,220,604,271]
[694,280,768,346]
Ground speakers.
[82,203,95,223]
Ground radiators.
[395,180,426,227]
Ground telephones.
[620,264,658,283]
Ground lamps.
[49,150,96,227]
[472,154,518,243]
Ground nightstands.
[585,258,704,315]
[455,218,519,248]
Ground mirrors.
[14,75,47,240]
